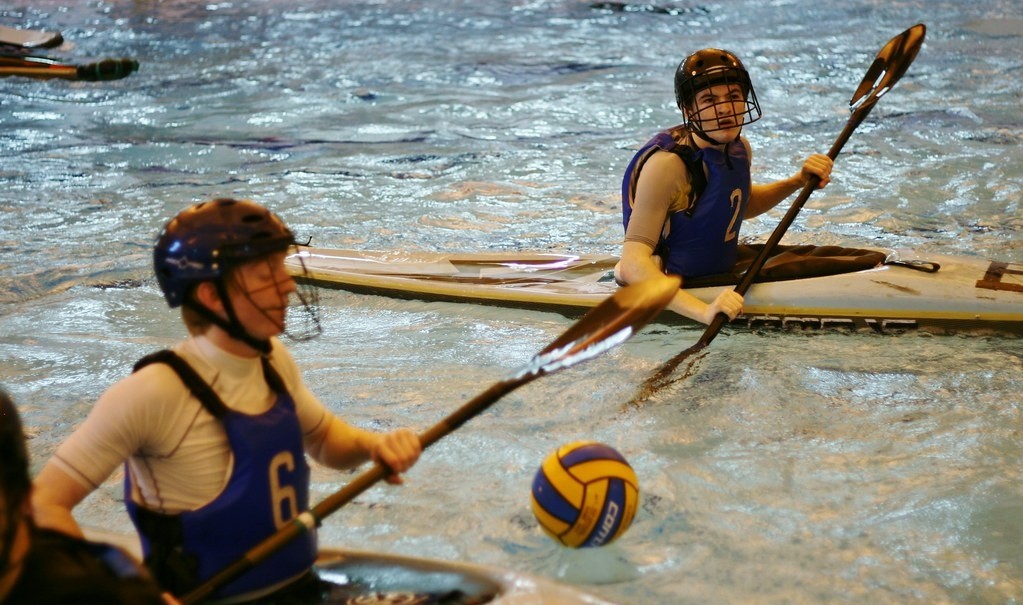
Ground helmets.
[153,199,293,308]
[675,49,752,109]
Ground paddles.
[697,23,928,346]
[178,273,683,605]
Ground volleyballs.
[529,440,640,549]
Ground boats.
[283,239,1022,323]
[0,26,140,80]
[77,525,613,605]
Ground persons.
[32,197,496,605]
[0,389,169,605]
[619,48,835,326]
